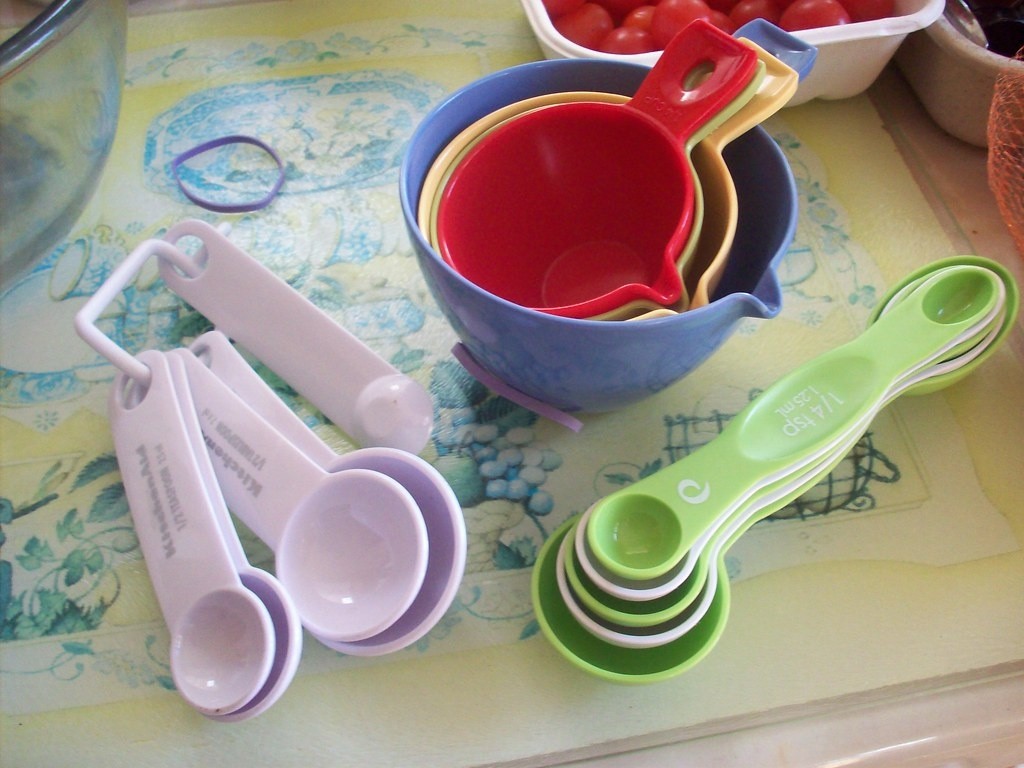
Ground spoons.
[531,253,1018,686]
[189,330,465,656]
[172,349,429,640]
[157,220,433,454]
[200,338,305,719]
[110,351,275,713]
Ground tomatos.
[539,0,896,56]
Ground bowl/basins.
[523,0,948,107]
[0,0,129,285]
[402,61,795,415]
